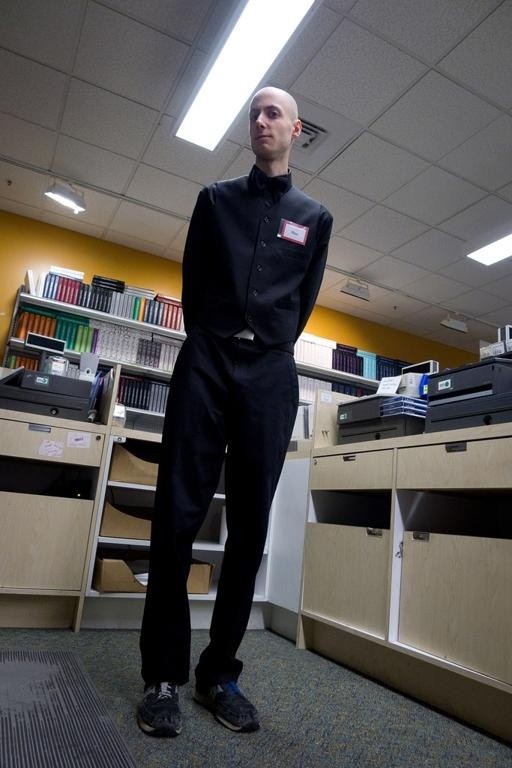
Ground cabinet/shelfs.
[1,281,384,415]
[0,402,111,631]
[102,361,172,432]
[292,435,395,712]
[81,424,274,635]
[374,422,511,750]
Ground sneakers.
[137,677,184,739]
[192,676,260,733]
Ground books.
[297,341,412,439]
[8,272,186,431]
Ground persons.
[135,86,332,738]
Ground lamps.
[41,172,87,216]
[338,271,373,301]
[438,307,470,333]
